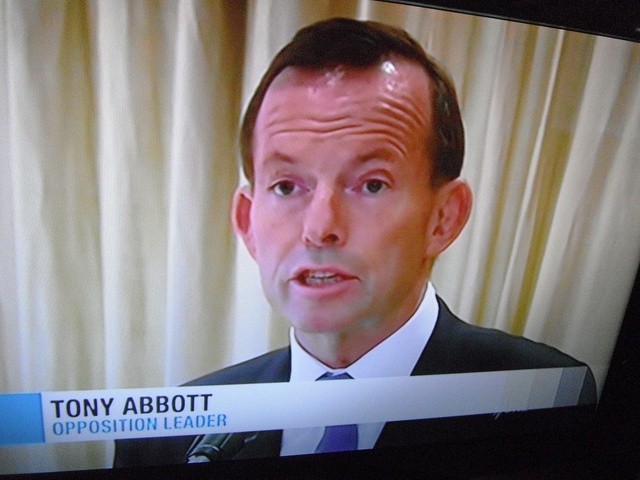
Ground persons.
[113,16,597,471]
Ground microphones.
[185,428,259,464]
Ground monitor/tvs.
[1,0,640,479]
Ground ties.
[313,374,358,454]
